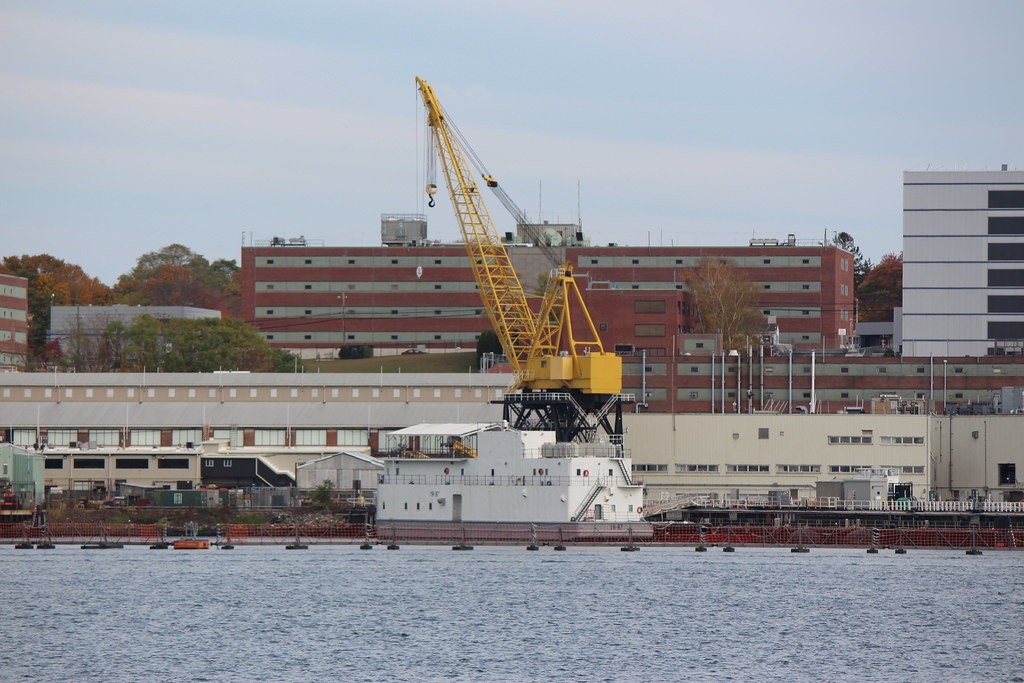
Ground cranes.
[412,74,637,449]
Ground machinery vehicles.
[391,444,431,459]
[439,439,477,457]
